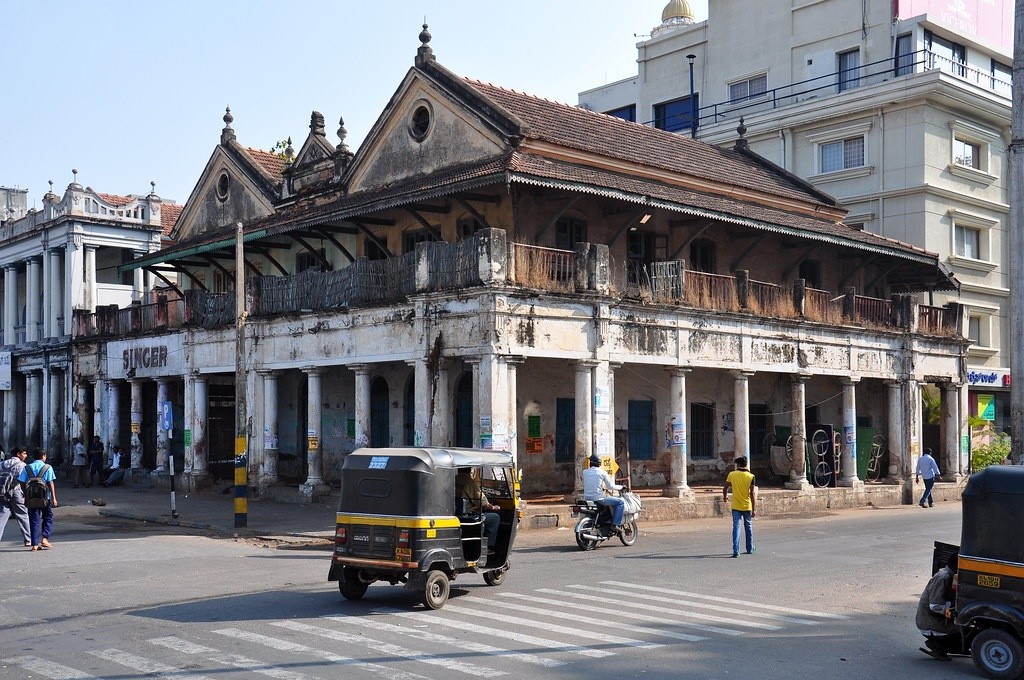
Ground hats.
[588,453,602,464]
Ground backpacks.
[0,460,24,503]
[23,463,51,511]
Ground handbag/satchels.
[613,487,642,524]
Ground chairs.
[456,498,485,534]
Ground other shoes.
[610,523,623,533]
[919,503,928,508]
[72,484,78,488]
[85,485,90,489]
[746,545,757,555]
[97,480,110,488]
[88,481,93,485]
[733,552,742,559]
[929,501,935,507]
[485,549,496,556]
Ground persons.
[456,468,501,555]
[89,436,105,485]
[17,447,57,551]
[581,455,625,532]
[723,457,756,558]
[102,446,130,487]
[73,438,89,488]
[0,445,31,547]
[915,448,942,508]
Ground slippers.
[41,541,53,548]
[918,647,953,661]
[30,545,42,552]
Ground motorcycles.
[328,447,525,610]
[932,465,1024,680]
[569,486,640,550]
